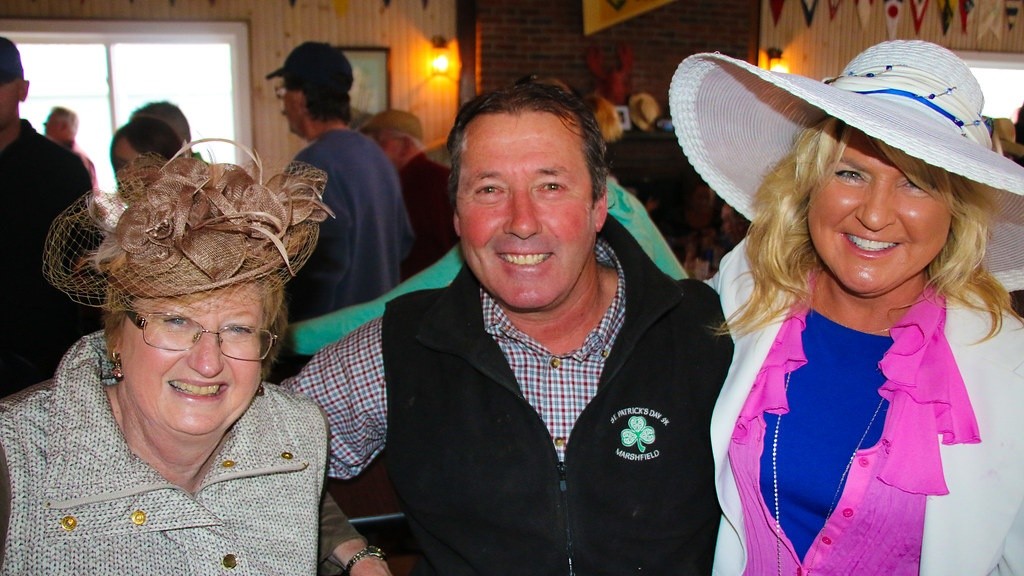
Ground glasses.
[119,294,277,361]
[275,86,287,99]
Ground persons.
[0,37,1024,576]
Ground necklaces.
[815,281,892,333]
[772,370,892,575]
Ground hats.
[358,110,422,139]
[670,40,1024,292]
[42,138,336,314]
[628,93,661,133]
[265,42,353,89]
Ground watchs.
[345,544,388,573]
